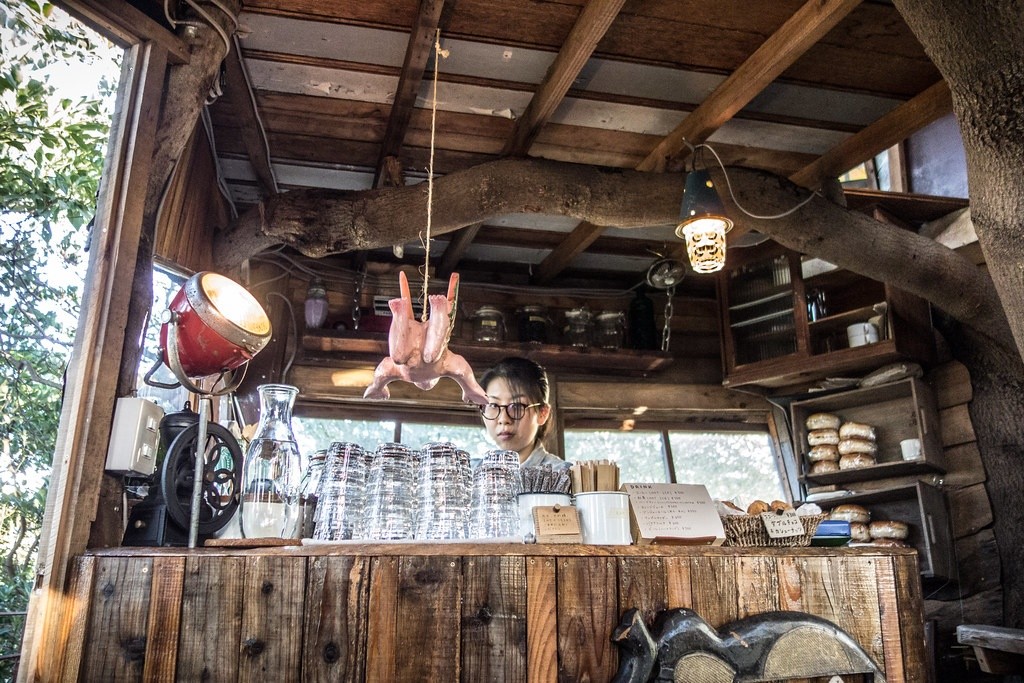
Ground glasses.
[480,403,542,420]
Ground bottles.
[239,383,302,539]
[517,302,550,344]
[562,309,594,348]
[470,303,508,343]
[596,311,625,350]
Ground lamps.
[144,269,273,390]
[674,169,733,274]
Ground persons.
[476,357,568,468]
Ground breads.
[748,412,909,543]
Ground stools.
[957,623,1023,674]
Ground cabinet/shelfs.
[796,483,955,576]
[285,263,674,371]
[788,377,946,480]
[721,205,941,397]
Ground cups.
[575,490,634,547]
[849,334,878,348]
[516,491,570,544]
[310,443,523,540]
[847,322,878,337]
